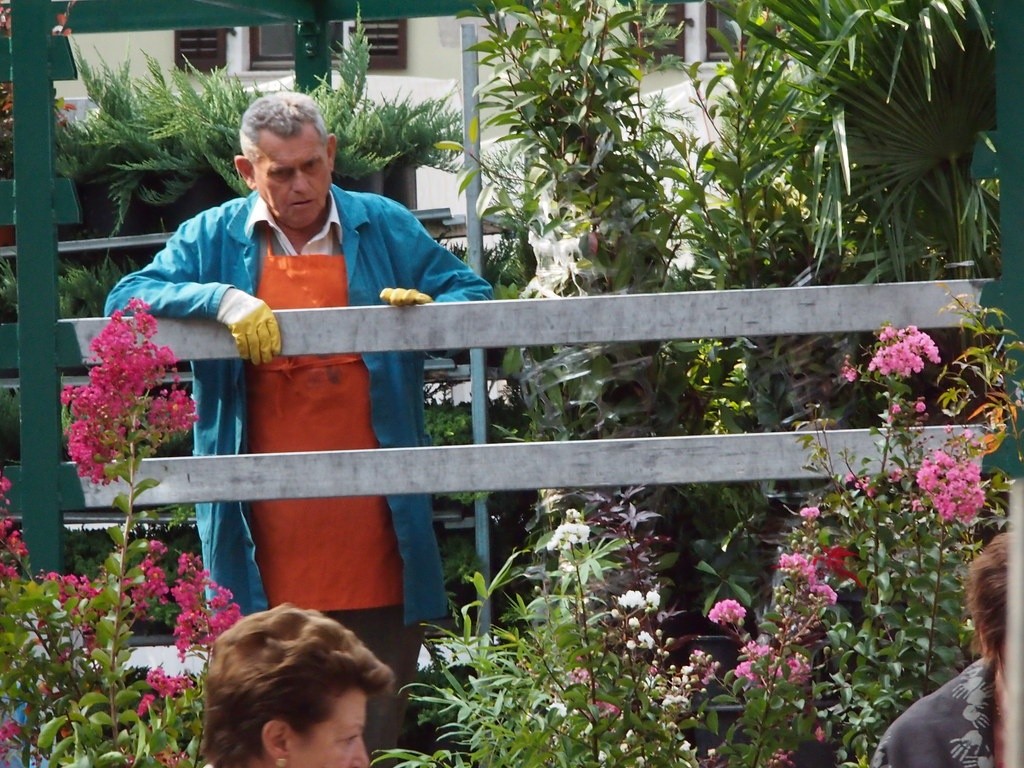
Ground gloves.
[380,287,434,306]
[216,286,282,365]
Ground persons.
[203,603,393,768]
[102,93,494,768]
[869,533,1008,768]
